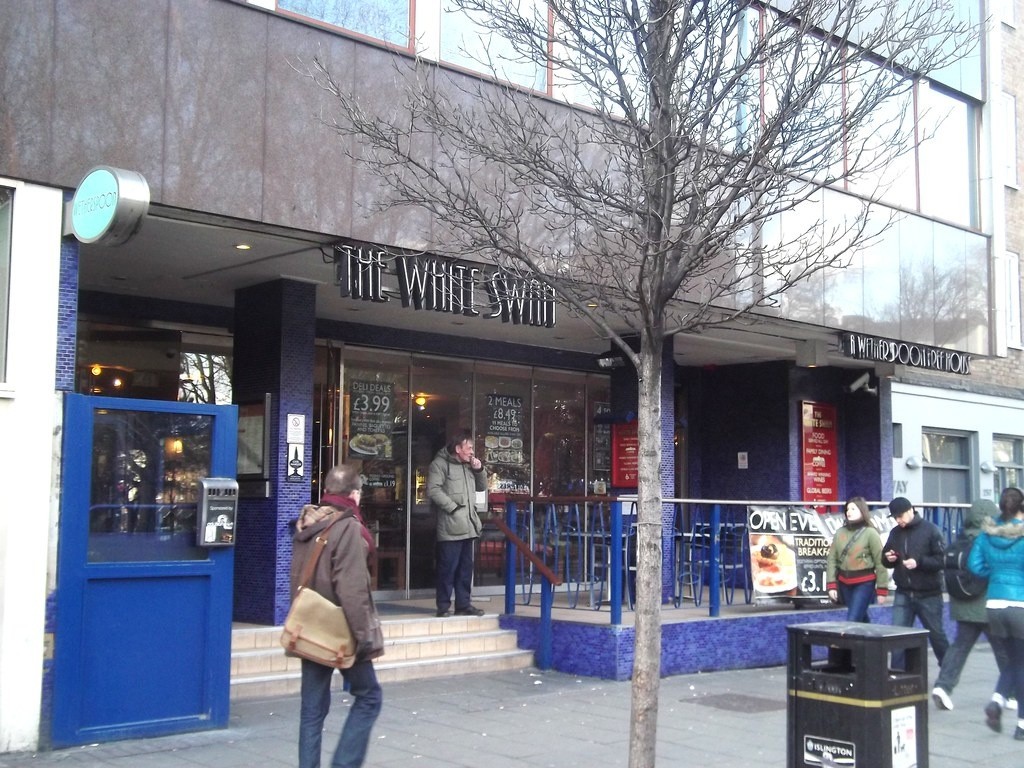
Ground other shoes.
[1013,725,1024,739]
[985,699,1005,732]
[1004,698,1018,711]
[931,687,953,711]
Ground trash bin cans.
[785,621,930,768]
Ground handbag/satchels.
[280,520,367,670]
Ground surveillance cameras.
[849,373,870,392]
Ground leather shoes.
[454,605,484,616]
[436,610,450,618]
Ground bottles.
[489,468,585,497]
[588,383,611,496]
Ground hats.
[886,497,911,519]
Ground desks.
[561,530,625,610]
[674,532,710,599]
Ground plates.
[485,436,524,464]
[349,433,390,455]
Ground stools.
[367,545,405,590]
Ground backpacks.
[942,526,988,600]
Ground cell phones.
[891,553,899,556]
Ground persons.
[931,499,1018,710]
[291,466,385,768]
[967,487,1024,740]
[827,497,888,624]
[427,433,488,617]
[881,497,949,678]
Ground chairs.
[694,521,744,603]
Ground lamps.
[415,398,427,412]
